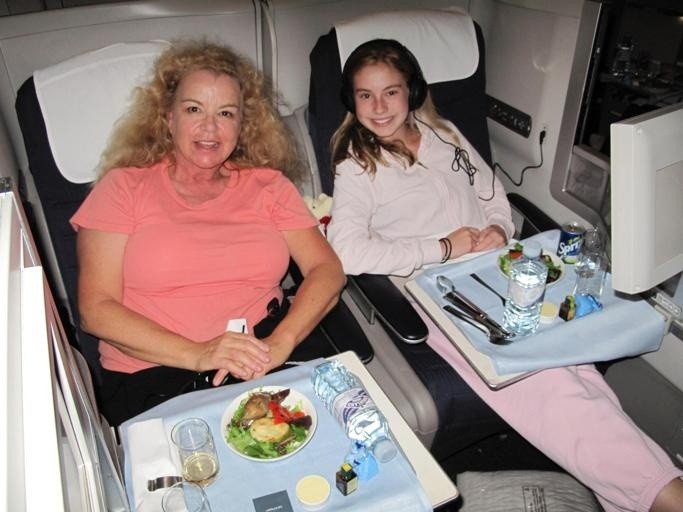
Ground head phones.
[339,39,428,111]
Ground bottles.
[310,360,397,463]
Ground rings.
[474,240,479,246]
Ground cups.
[160,480,213,512]
[169,417,221,488]
[570,249,611,303]
[582,228,609,258]
[502,256,549,338]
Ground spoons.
[434,272,516,344]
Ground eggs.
[521,240,542,259]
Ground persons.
[325,39,683,511]
[66,44,347,446]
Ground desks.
[405,230,671,391]
[116,350,459,512]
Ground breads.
[250,418,290,442]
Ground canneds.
[557,222,585,266]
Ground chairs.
[308,8,560,454]
[14,36,417,512]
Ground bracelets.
[438,239,448,261]
[440,236,452,264]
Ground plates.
[497,246,566,288]
[218,385,319,462]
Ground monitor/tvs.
[611,103,683,295]
